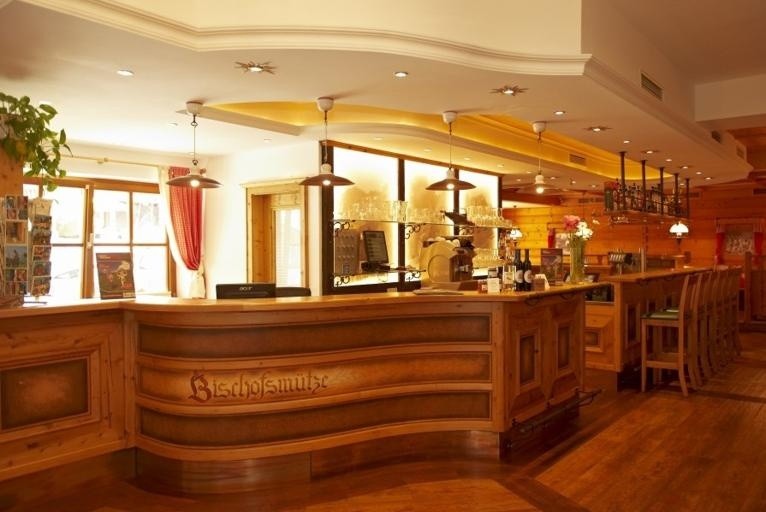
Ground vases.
[565,240,593,282]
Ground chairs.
[640,264,745,395]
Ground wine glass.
[459,206,513,227]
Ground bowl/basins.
[444,236,473,246]
[608,252,631,263]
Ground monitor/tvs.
[216,283,276,299]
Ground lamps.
[669,170,692,238]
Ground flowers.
[563,215,593,240]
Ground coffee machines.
[419,242,478,289]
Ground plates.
[432,281,462,290]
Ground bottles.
[441,210,475,226]
[360,262,391,271]
[522,249,532,291]
[602,178,684,215]
[513,249,523,291]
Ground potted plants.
[0,93,74,196]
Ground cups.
[630,247,647,274]
[555,267,564,285]
[673,255,685,270]
[584,275,593,283]
[478,263,517,293]
[344,196,446,225]
